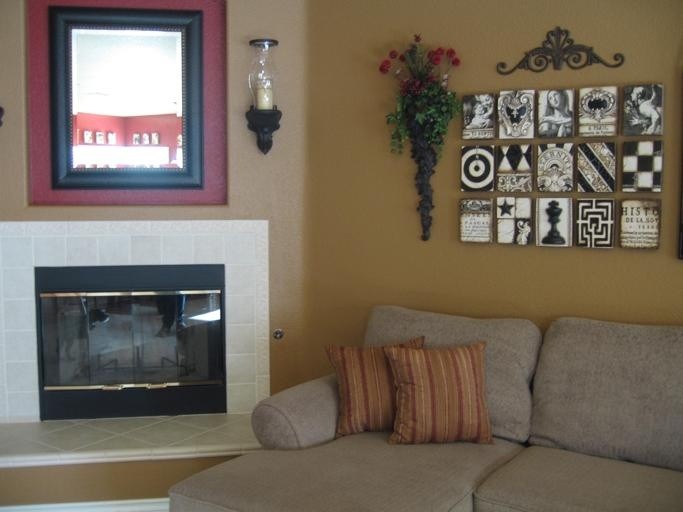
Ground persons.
[466,93,494,129]
[626,86,662,136]
[154,294,186,339]
[538,90,572,136]
[54,295,91,365]
[471,104,487,126]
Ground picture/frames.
[22,0,231,206]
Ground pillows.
[321,333,494,446]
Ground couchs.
[165,306,681,510]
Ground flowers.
[377,31,464,165]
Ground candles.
[255,79,273,111]
